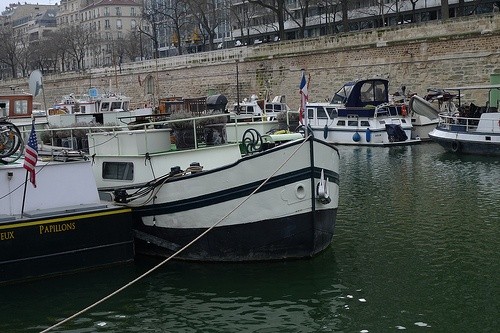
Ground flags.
[23,120,39,188]
[298,74,308,124]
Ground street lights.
[234,57,241,116]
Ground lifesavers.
[450,140,459,152]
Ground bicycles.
[0,102,26,166]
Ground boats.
[0,91,232,148]
[1,146,133,264]
[85,123,341,261]
[223,95,299,144]
[297,78,454,144]
[428,84,500,157]
